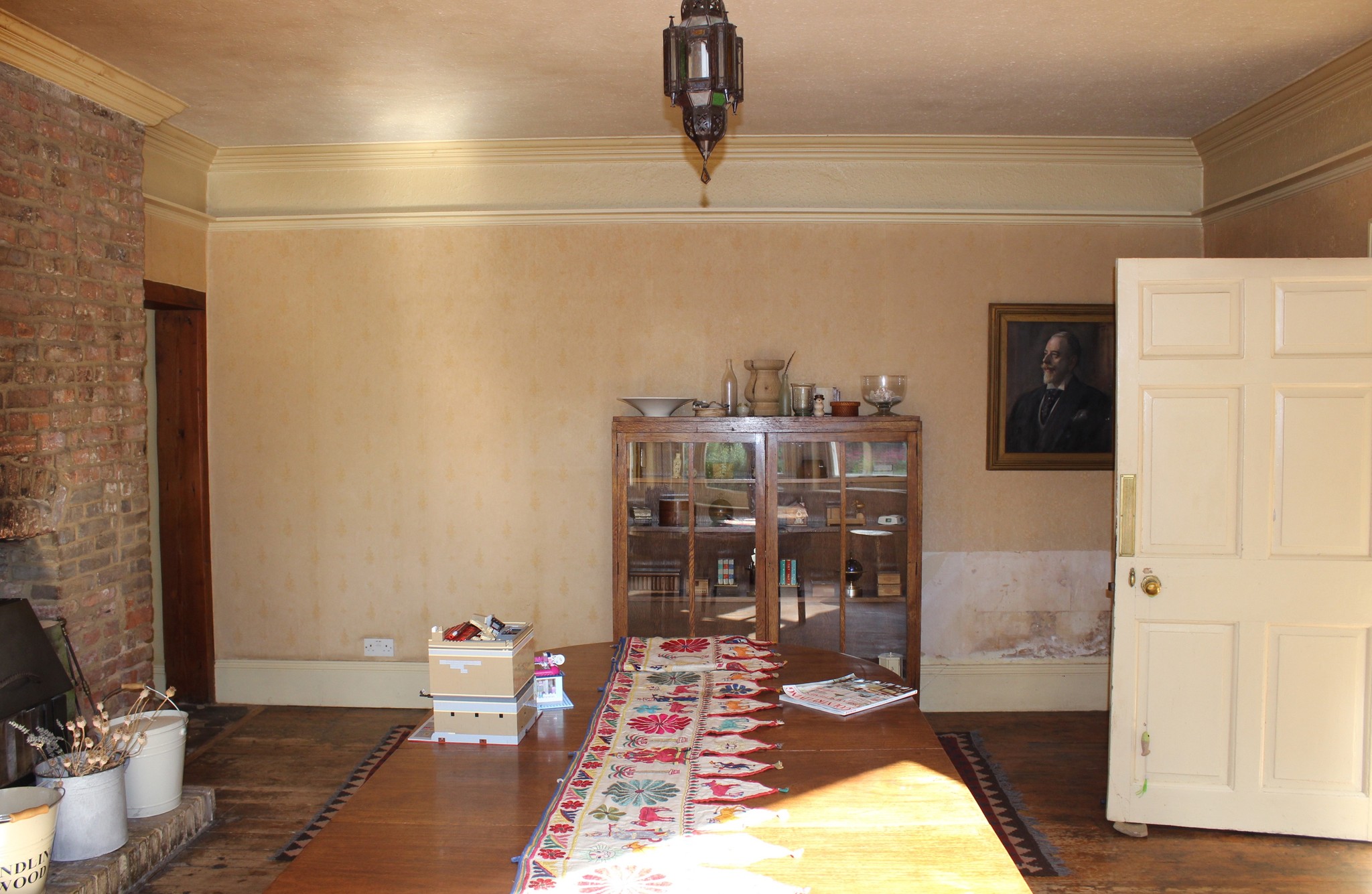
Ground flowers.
[9,685,177,777]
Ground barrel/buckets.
[32,749,130,861]
[0,786,65,894]
[93,683,189,818]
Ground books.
[717,558,736,586]
[779,672,918,717]
[780,558,799,586]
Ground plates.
[616,397,697,417]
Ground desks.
[265,634,1034,894]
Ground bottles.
[779,374,791,416]
[737,403,750,417]
[720,359,737,417]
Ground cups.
[790,383,816,416]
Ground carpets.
[270,725,415,862]
[935,730,1071,877]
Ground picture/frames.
[986,303,1114,471]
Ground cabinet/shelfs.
[612,415,922,714]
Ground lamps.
[663,0,744,183]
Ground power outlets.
[364,639,393,657]
